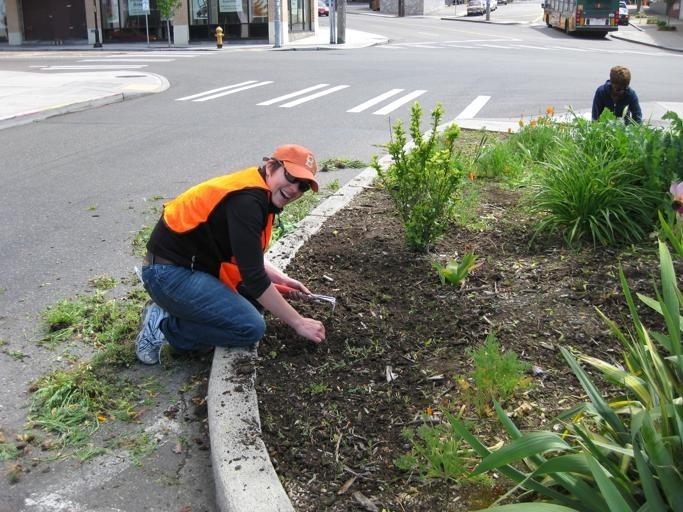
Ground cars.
[467,0,507,16]
[106,27,159,41]
[619,2,629,26]
[318,1,329,16]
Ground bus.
[541,0,619,38]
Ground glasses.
[271,157,311,192]
[614,85,630,92]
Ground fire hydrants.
[214,26,224,48]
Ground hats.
[271,143,320,193]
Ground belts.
[142,250,176,268]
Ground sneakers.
[135,298,172,364]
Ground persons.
[132,144,329,367]
[588,63,641,127]
